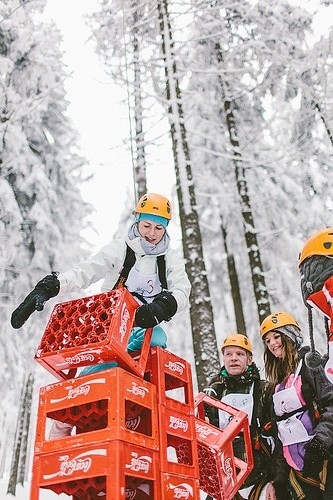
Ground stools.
[30,286,254,500]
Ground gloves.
[11,275,60,329]
[302,438,327,478]
[135,291,177,329]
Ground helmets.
[222,333,252,354]
[298,227,333,267]
[259,313,301,339]
[135,193,173,219]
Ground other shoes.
[48,420,72,440]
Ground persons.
[11,192,192,443]
[298,227,333,384]
[199,312,333,499]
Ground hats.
[300,255,333,370]
